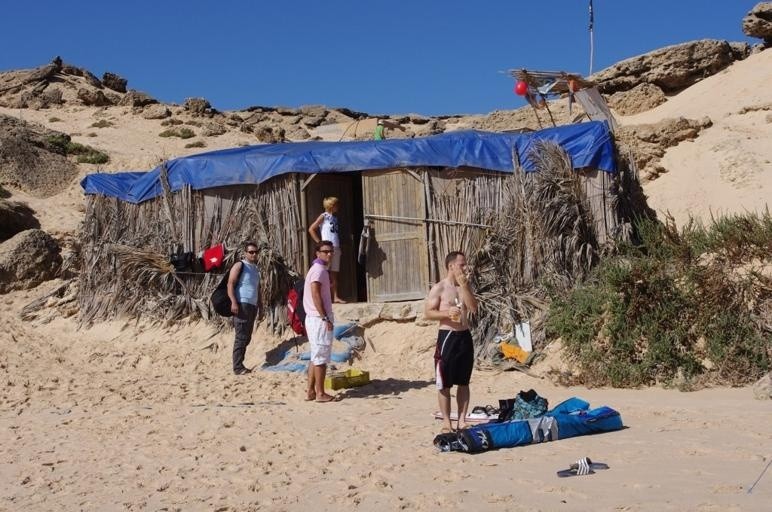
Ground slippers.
[441,424,471,433]
[305,397,340,403]
[557,457,607,478]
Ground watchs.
[322,315,331,322]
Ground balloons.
[514,80,527,95]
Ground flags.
[202,243,224,272]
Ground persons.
[302,239,342,403]
[425,250,479,433]
[306,196,347,305]
[373,119,387,140]
[226,243,265,375]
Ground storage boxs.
[324,368,369,391]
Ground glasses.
[319,250,333,254]
[246,250,259,254]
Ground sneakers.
[234,369,251,375]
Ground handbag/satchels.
[287,280,306,335]
[512,389,547,419]
[210,271,234,317]
